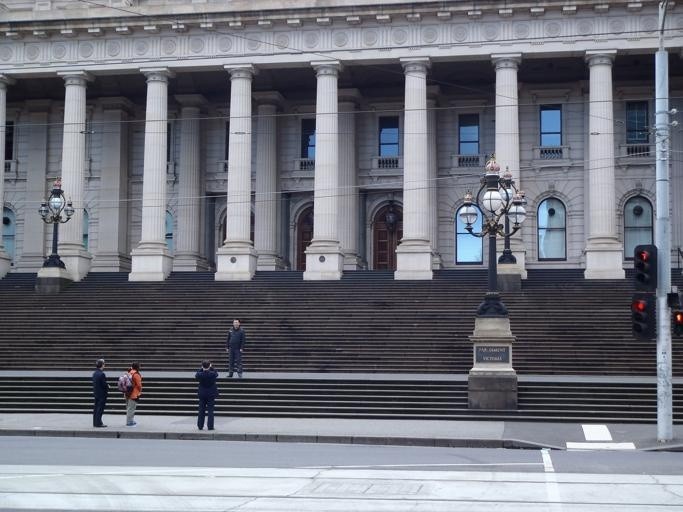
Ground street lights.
[496,165,527,291]
[34,176,73,291]
[459,153,529,409]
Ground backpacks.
[118,372,138,396]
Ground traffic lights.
[672,310,682,336]
[630,291,655,341]
[632,245,657,292]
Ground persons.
[92,359,109,427]
[195,361,219,429]
[125,361,142,425]
[227,319,245,380]
